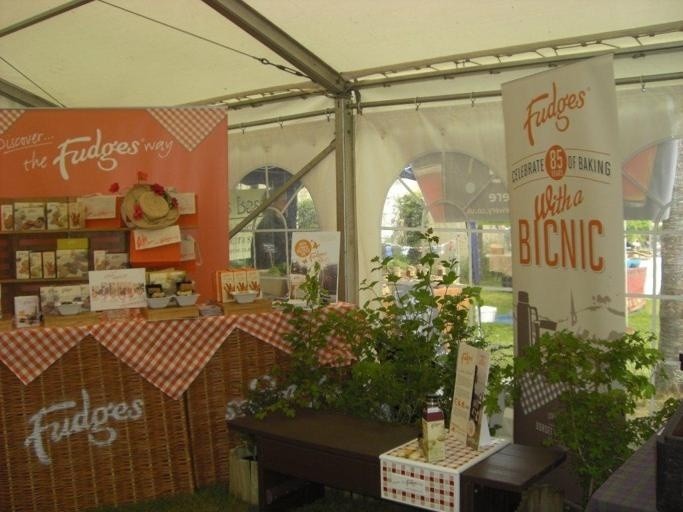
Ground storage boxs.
[130,230,182,269]
[78,195,126,228]
[260,272,287,297]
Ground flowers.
[108,169,181,221]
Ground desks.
[586,430,682,512]
[226,407,567,512]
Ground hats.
[123,183,180,229]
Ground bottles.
[421,394,444,463]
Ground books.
[448,341,496,455]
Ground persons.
[250,185,290,270]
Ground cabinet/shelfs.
[1,225,129,285]
[42,310,99,326]
[1,311,14,331]
[216,297,272,316]
[140,305,198,321]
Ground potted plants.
[231,380,294,507]
